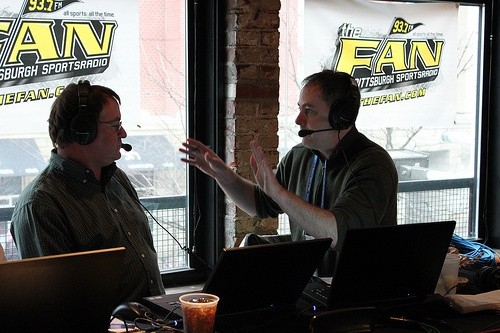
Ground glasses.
[97,120,122,135]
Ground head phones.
[69,80,98,144]
[327,72,360,131]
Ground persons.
[180,70,398,277]
[10,81,165,313]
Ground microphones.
[297,128,336,137]
[121,143,132,151]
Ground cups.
[435,253,460,300]
[179,292,220,333]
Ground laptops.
[1,246,125,333]
[112,301,155,323]
[302,220,457,312]
[142,237,333,329]
